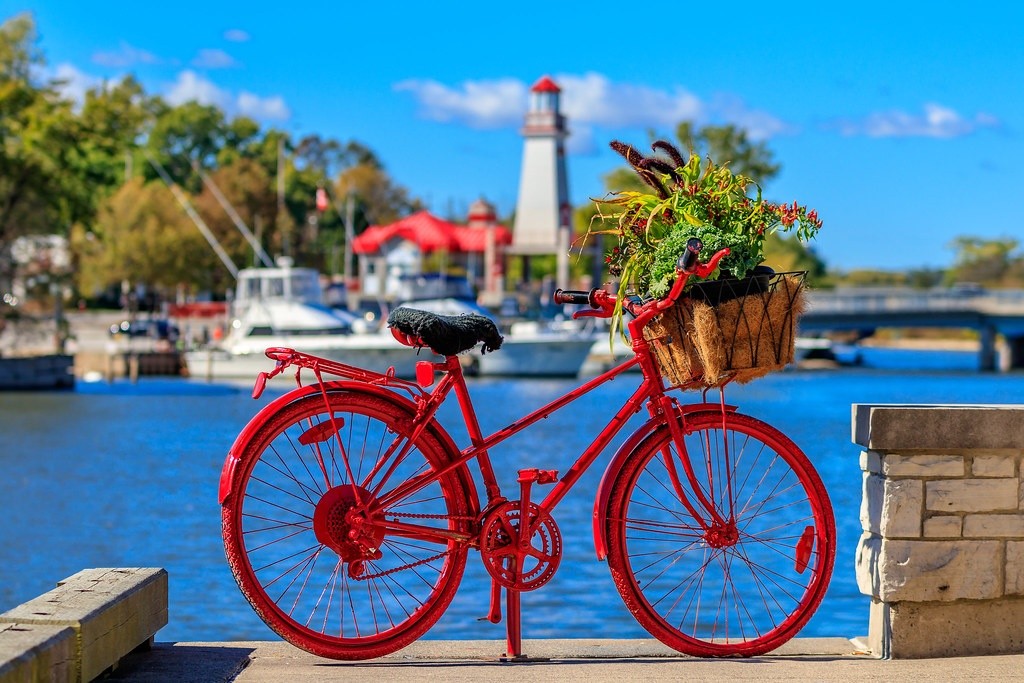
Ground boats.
[180,239,830,390]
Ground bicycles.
[218,238,838,662]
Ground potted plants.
[566,141,824,360]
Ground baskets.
[621,271,808,392]
